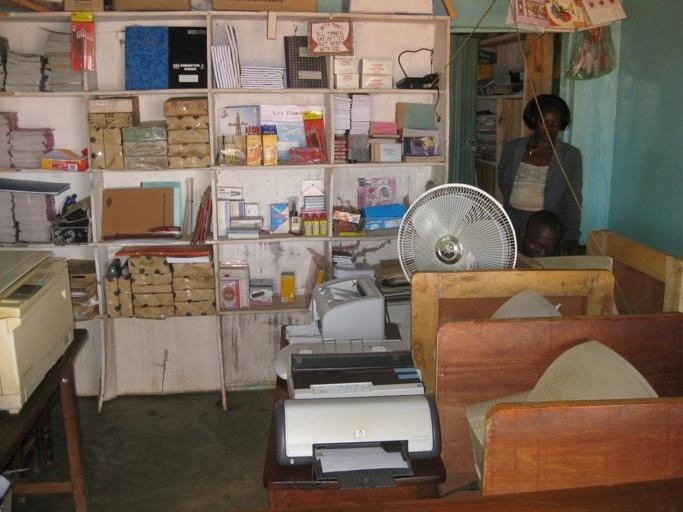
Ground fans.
[399,181,518,288]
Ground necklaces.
[526,137,546,157]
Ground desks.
[1,330,91,510]
[262,322,448,511]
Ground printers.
[285,273,387,347]
[263,398,447,486]
[287,341,425,400]
[0,250,75,415]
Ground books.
[0,24,84,93]
[123,25,169,90]
[0,178,71,243]
[332,91,448,163]
[331,250,413,304]
[0,110,54,171]
[166,25,207,90]
[283,34,329,88]
[209,23,285,88]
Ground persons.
[494,92,582,243]
[514,209,587,260]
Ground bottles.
[302,213,328,236]
[107,257,121,281]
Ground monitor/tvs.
[463,340,661,490]
[491,286,562,318]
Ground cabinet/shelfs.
[474,31,554,205]
[2,12,454,411]
